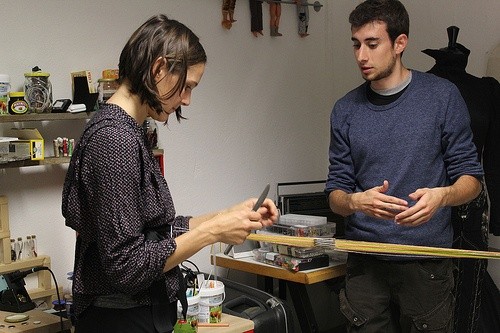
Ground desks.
[194,312,256,332]
[211,249,349,331]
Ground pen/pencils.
[188,279,203,296]
[205,271,217,288]
[197,323,228,327]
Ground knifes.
[225,184,271,255]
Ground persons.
[296,0,310,38]
[267,0,283,36]
[62,14,280,333]
[249,0,264,38]
[222,0,237,29]
[324,0,486,333]
[422,26,500,333]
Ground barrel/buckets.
[199,280,225,323]
[177,288,198,324]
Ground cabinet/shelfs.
[1,195,77,331]
[0,112,163,168]
